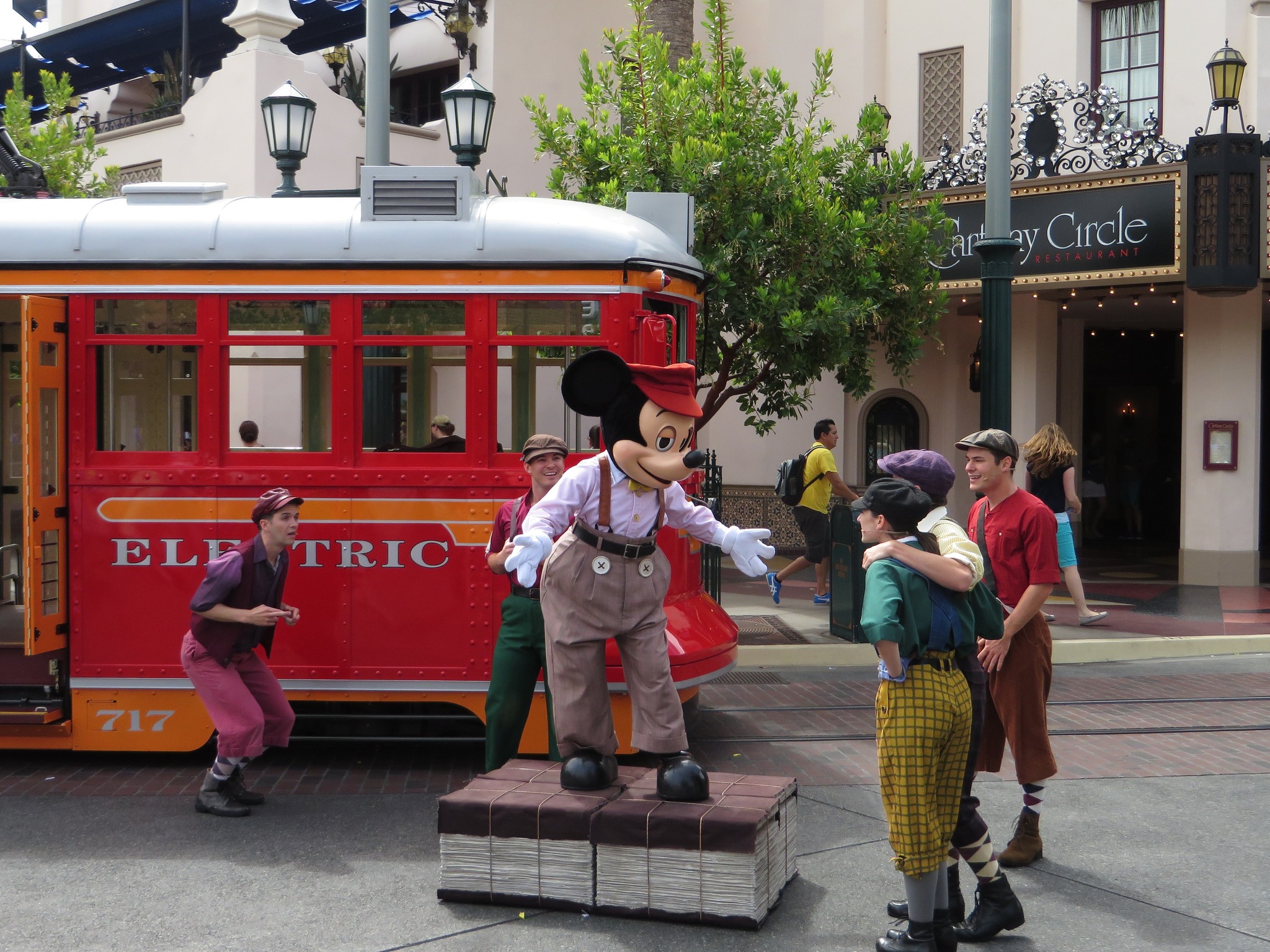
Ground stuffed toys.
[503,348,775,804]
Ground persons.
[179,488,304,817]
[765,418,859,604]
[862,449,1025,943]
[587,424,601,449]
[483,434,564,772]
[852,477,1004,952]
[1018,422,1108,626]
[431,415,455,439]
[239,421,264,448]
[956,429,1061,869]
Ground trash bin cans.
[827,503,870,643]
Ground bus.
[0,167,739,753]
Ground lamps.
[969,336,980,393]
[64,95,89,112]
[321,43,354,89]
[413,0,488,71]
[150,73,166,97]
[1195,38,1255,136]
[861,94,891,171]
[32,5,46,20]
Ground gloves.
[876,655,913,683]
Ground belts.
[911,658,959,672]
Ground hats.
[878,449,955,498]
[251,488,305,523]
[432,416,450,429]
[851,477,932,524]
[953,428,1019,466]
[522,434,570,464]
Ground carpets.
[1100,571,1157,578]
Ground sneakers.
[813,592,831,605]
[766,572,782,604]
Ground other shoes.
[1079,611,1108,626]
[1038,609,1056,621]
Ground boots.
[195,771,252,818]
[875,921,959,952]
[886,861,965,921]
[950,873,1026,942]
[998,808,1043,867]
[224,768,264,804]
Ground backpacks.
[774,446,829,507]
